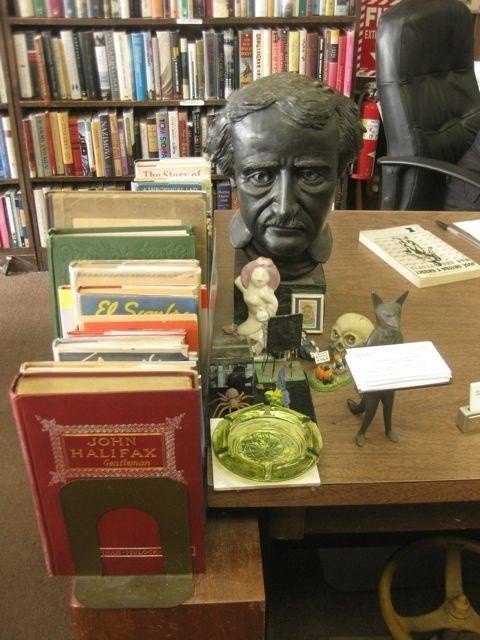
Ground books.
[358,223,480,289]
[12,23,356,101]
[9,191,211,576]
[0,186,125,250]
[0,109,227,179]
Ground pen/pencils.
[436,218,480,250]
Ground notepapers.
[343,339,453,395]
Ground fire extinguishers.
[348,88,381,182]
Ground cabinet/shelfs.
[0,33,37,271]
[0,1,361,272]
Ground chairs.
[376,0,480,211]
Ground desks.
[206,209,480,594]
[69,510,266,640]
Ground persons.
[203,73,367,325]
[10,1,354,18]
[234,257,282,353]
